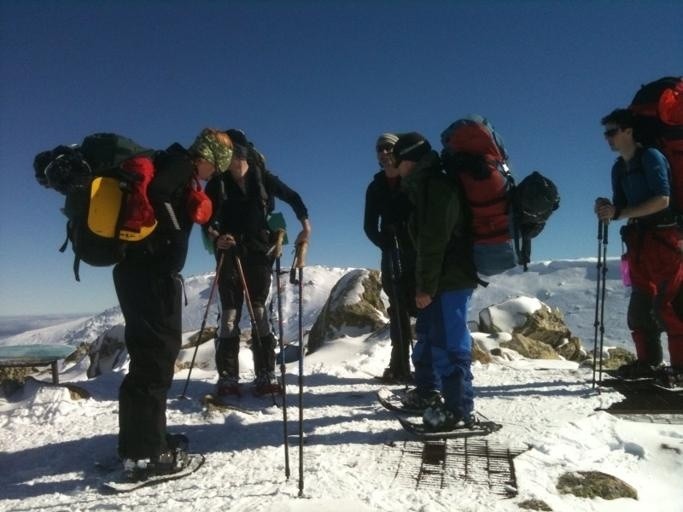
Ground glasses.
[604,129,618,137]
[376,142,393,152]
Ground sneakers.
[618,359,683,387]
[262,384,281,397]
[218,382,241,399]
[384,368,475,431]
[116,434,192,482]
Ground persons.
[200,130,311,398]
[393,132,478,430]
[594,109,683,393]
[364,133,418,380]
[111,127,234,482]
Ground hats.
[393,132,432,168]
[226,128,247,146]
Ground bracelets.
[613,204,621,221]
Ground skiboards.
[595,363,683,392]
[376,386,501,440]
[200,377,290,412]
[94,430,205,494]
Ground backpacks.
[33,134,147,281]
[441,115,516,276]
[628,77,683,206]
